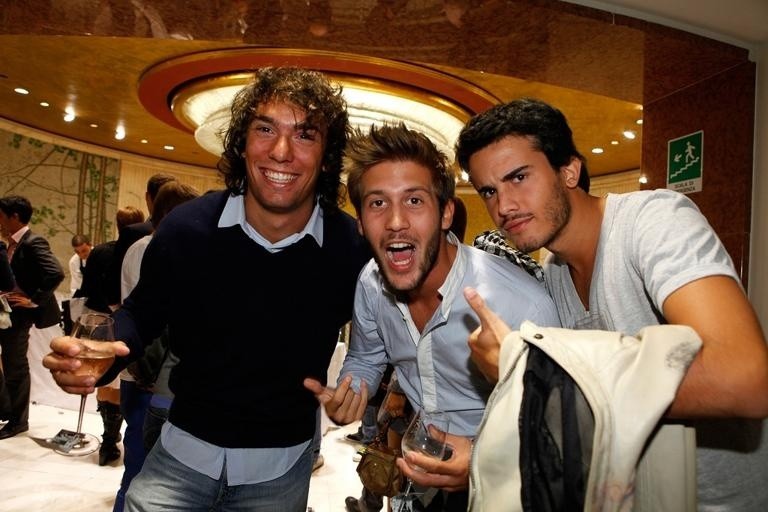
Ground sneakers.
[344,434,360,442]
[353,446,366,462]
[312,455,324,471]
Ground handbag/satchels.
[356,413,409,499]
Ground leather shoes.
[345,496,358,512]
[0,422,29,440]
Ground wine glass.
[389,408,451,512]
[53,313,115,457]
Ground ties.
[7,244,17,265]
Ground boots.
[97,401,121,444]
[99,409,124,466]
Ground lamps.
[170,73,479,181]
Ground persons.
[346,380,414,512]
[0,239,17,292]
[120,173,175,255]
[142,353,179,463]
[454,99,767,512]
[347,363,394,445]
[0,196,65,440]
[83,205,145,466]
[44,66,374,512]
[114,182,200,512]
[69,237,94,296]
[306,126,561,512]
